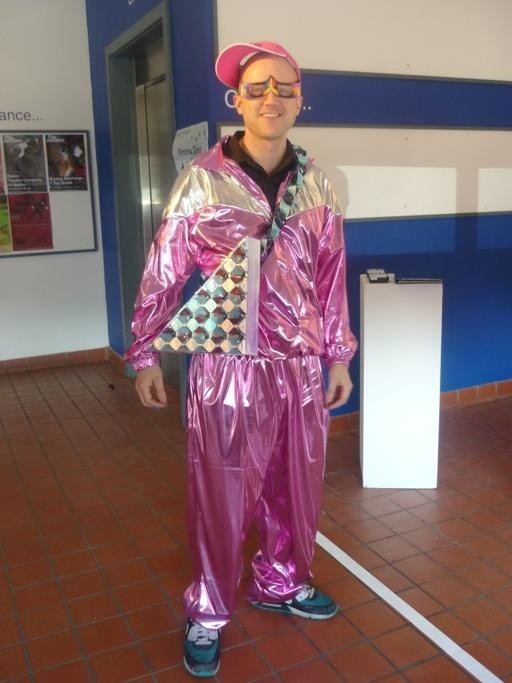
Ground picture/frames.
[0,126,100,259]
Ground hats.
[215,41,301,89]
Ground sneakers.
[183,616,220,677]
[251,585,337,619]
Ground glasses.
[244,75,299,98]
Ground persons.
[29,196,49,222]
[115,38,360,679]
[3,137,40,176]
[54,146,80,177]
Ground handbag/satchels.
[141,236,259,353]
